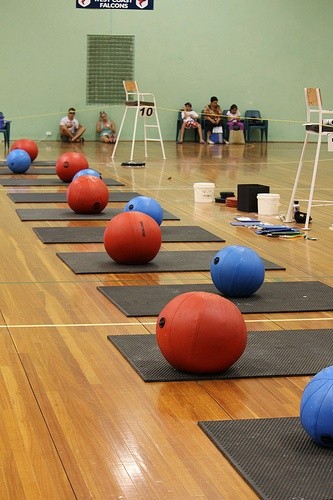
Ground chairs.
[176,109,268,143]
[123,80,156,108]
[0,112,12,149]
[305,87,333,135]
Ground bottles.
[293,201,300,219]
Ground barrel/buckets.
[194,182,215,203]
[257,193,281,215]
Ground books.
[230,217,301,239]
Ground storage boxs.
[238,184,270,213]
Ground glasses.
[69,112,75,115]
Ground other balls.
[7,139,39,174]
[104,195,163,265]
[300,365,333,450]
[156,291,247,374]
[210,245,266,297]
[55,152,109,216]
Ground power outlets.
[46,132,51,135]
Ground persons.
[96,111,116,143]
[176,102,206,144]
[60,108,85,143]
[226,104,244,132]
[203,96,230,144]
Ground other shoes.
[68,137,71,143]
[79,138,84,142]
[207,139,215,144]
[200,140,206,144]
[110,136,116,142]
[178,140,183,143]
[224,139,229,144]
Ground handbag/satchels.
[229,129,246,144]
